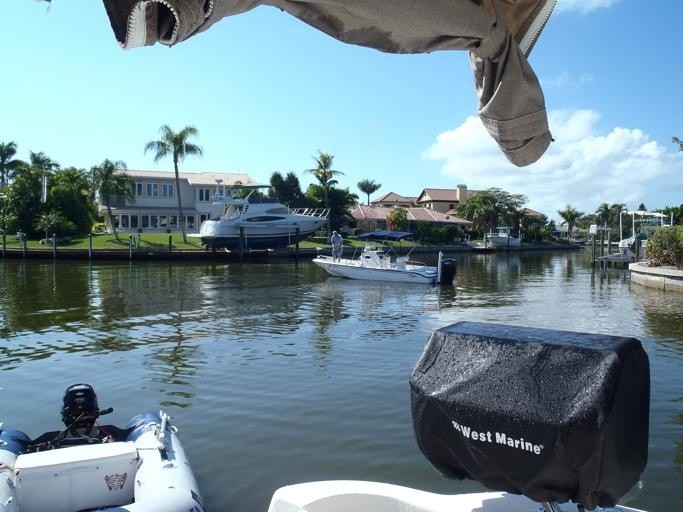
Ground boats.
[186,184,331,250]
[312,232,456,283]
[486,226,522,247]
[0,383,206,512]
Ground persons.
[330,229,344,263]
